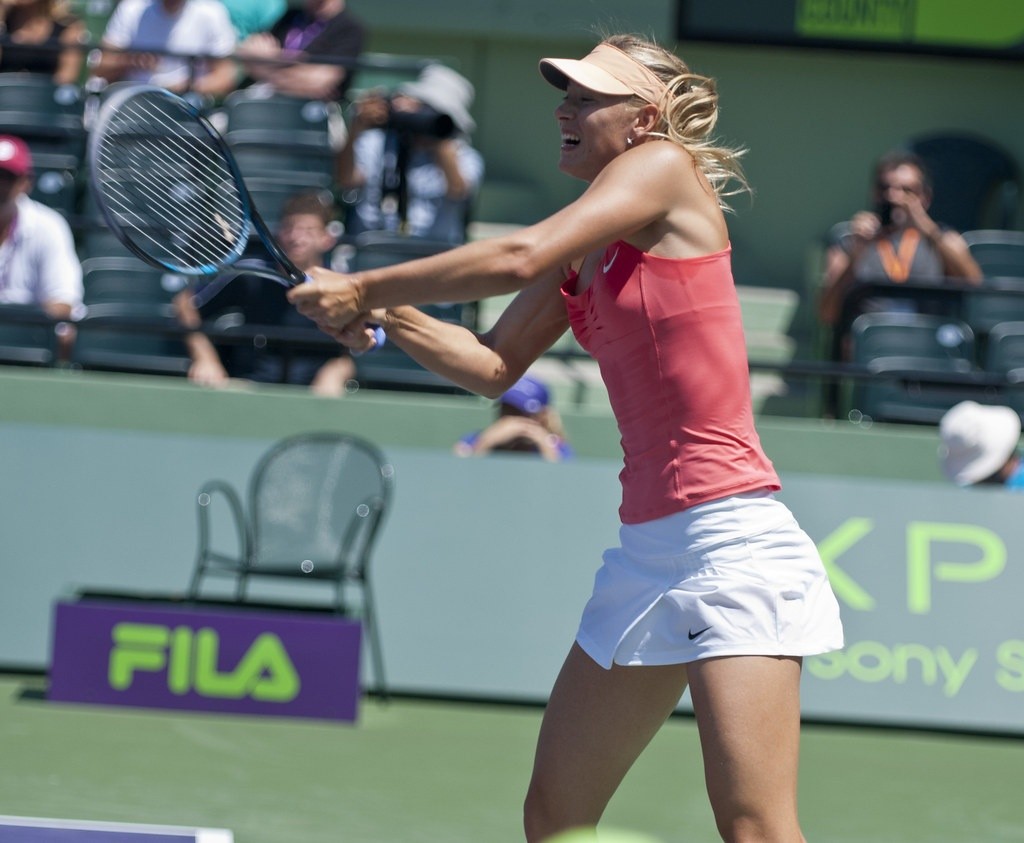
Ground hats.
[400,64,477,133]
[936,400,1021,488]
[494,380,549,416]
[539,42,675,110]
[0,135,30,177]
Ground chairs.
[2,46,483,397]
[821,211,1024,432]
[188,430,398,703]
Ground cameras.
[373,97,455,138]
[866,200,899,226]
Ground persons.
[452,372,572,461]
[327,62,485,247]
[171,188,371,393]
[934,397,1023,490]
[815,150,984,415]
[0,135,89,364]
[0,2,365,105]
[288,25,846,842]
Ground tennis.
[85,86,388,360]
